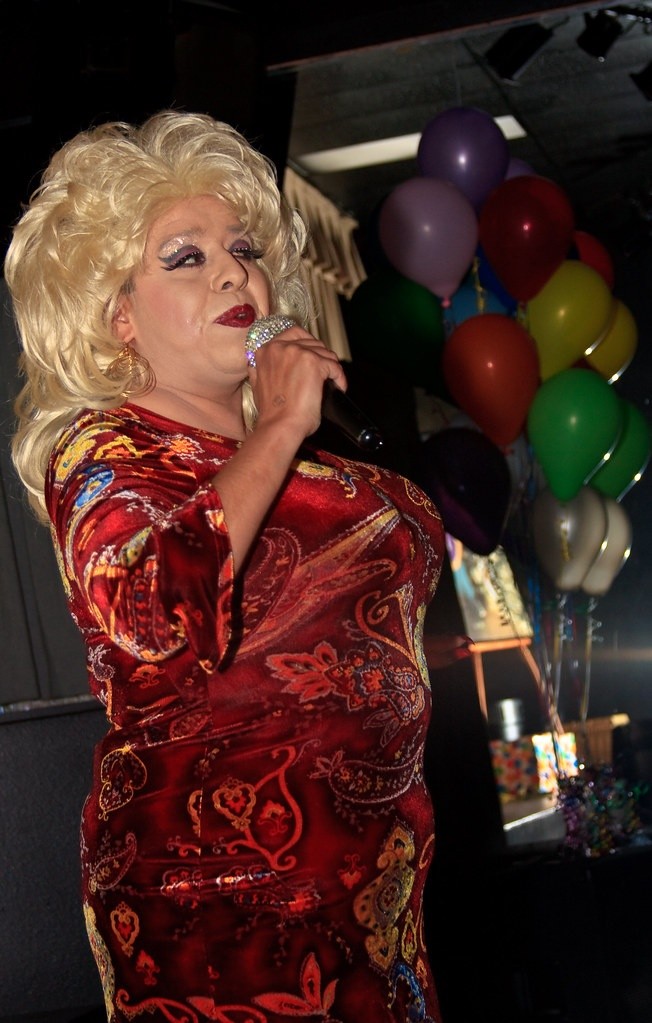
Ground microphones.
[243,313,384,455]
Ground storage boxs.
[494,712,630,805]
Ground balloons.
[352,106,652,595]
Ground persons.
[0,108,448,1023]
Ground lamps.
[485,22,554,81]
[577,10,622,60]
